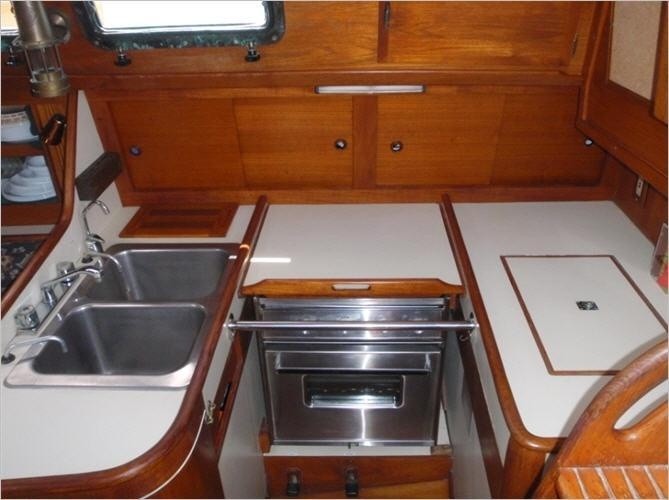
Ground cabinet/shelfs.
[233,84,508,188]
[3,64,69,219]
[81,70,243,209]
[380,3,599,76]
[498,83,606,193]
[194,298,271,498]
[441,309,510,498]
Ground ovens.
[262,355,441,446]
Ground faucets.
[1,335,69,364]
[81,199,110,267]
[40,265,103,304]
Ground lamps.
[8,0,70,100]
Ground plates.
[2,156,57,204]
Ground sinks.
[85,247,230,300]
[32,302,208,376]
